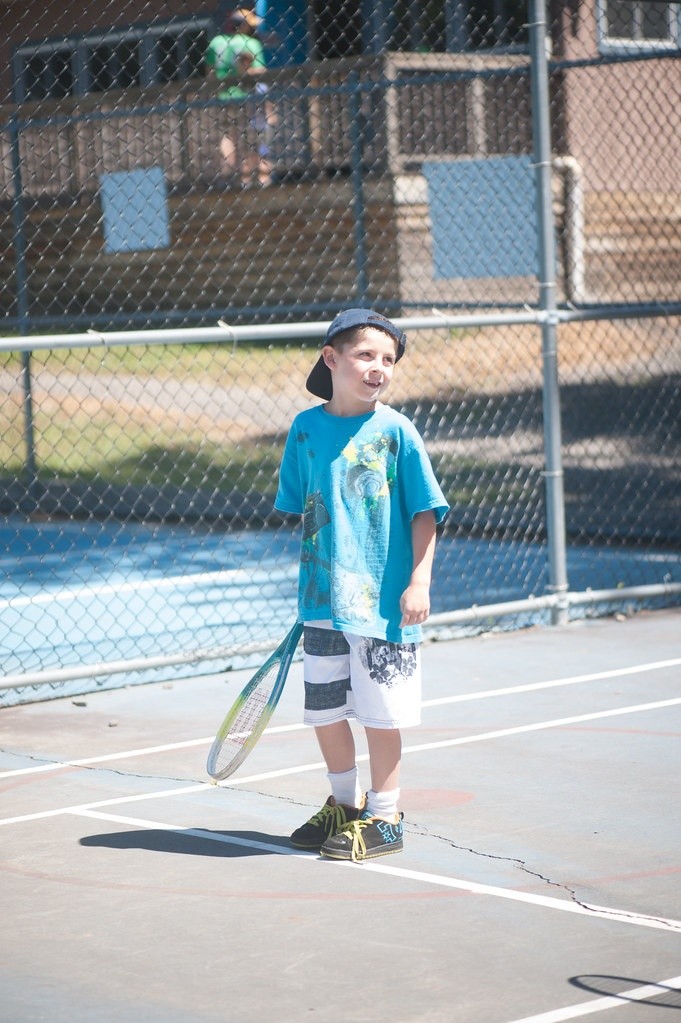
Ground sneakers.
[319,792,405,864]
[288,792,367,849]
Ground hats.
[307,308,407,401]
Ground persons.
[204,8,264,188]
[237,53,273,189]
[273,308,451,860]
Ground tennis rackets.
[206,615,305,781]
